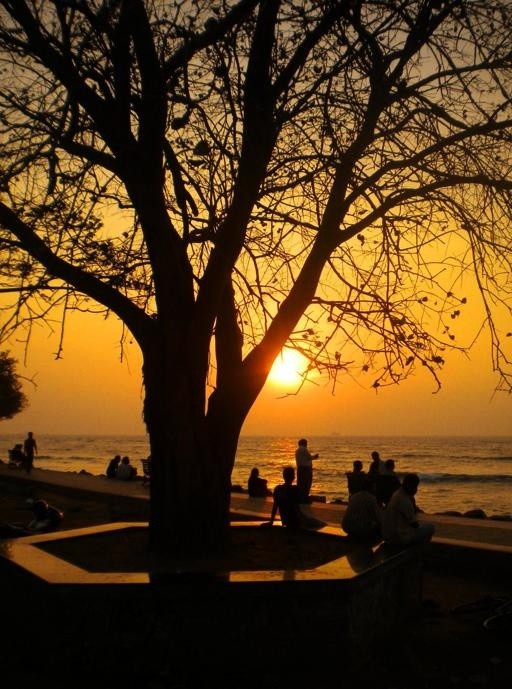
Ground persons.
[106,454,121,477]
[365,451,384,478]
[24,431,38,473]
[10,443,19,462]
[117,456,138,482]
[377,459,424,514]
[295,437,320,504]
[383,473,436,547]
[247,467,272,498]
[260,465,328,528]
[16,444,35,470]
[348,461,372,494]
[340,482,386,541]
